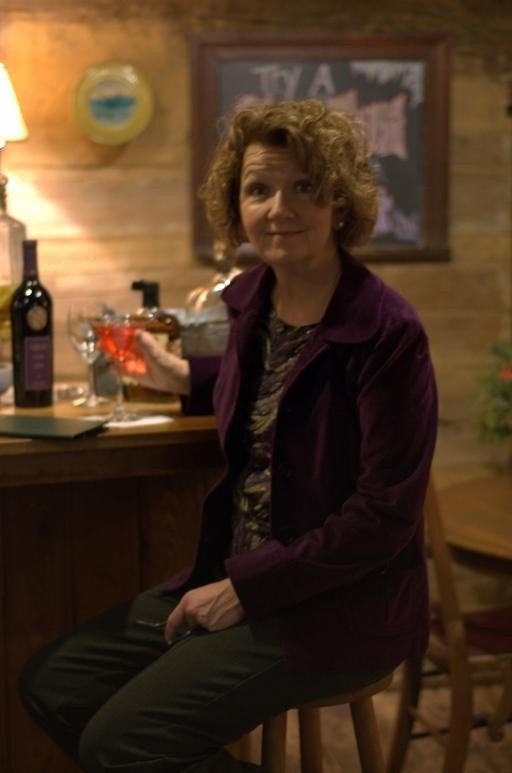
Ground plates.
[82,413,174,427]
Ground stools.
[258,672,393,773]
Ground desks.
[430,459,508,569]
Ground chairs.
[386,467,508,773]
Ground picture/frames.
[186,31,452,261]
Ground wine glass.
[67,298,110,405]
[87,304,156,422]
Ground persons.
[17,99,436,773]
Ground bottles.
[129,284,179,403]
[0,174,28,356]
[10,239,54,404]
